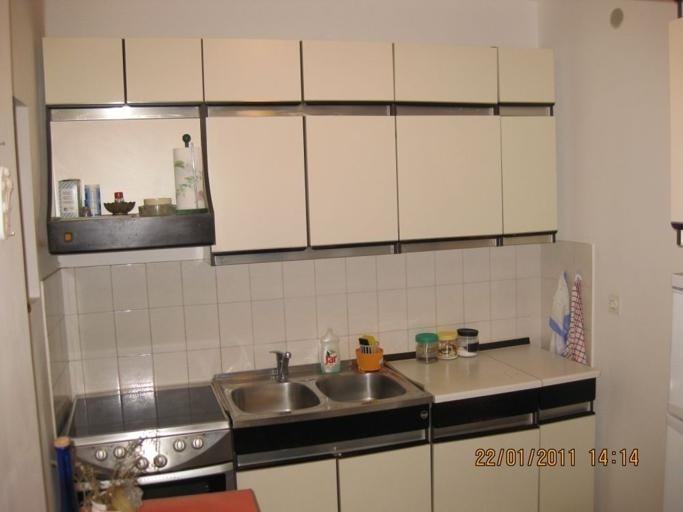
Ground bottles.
[414,327,480,363]
[112,191,124,203]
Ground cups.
[354,347,384,372]
[82,183,101,216]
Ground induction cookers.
[62,381,233,483]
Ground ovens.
[69,461,236,506]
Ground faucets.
[269,349,292,382]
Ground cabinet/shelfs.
[392,43,557,107]
[399,116,559,242]
[432,414,540,512]
[539,410,598,512]
[205,36,393,106]
[39,35,202,108]
[237,442,434,511]
[204,115,396,256]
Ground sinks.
[314,371,407,404]
[229,381,322,415]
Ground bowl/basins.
[103,200,136,215]
[143,197,171,216]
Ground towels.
[547,271,570,358]
[565,281,588,364]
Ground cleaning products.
[319,329,342,376]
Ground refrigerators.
[660,270,682,512]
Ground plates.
[137,204,177,218]
